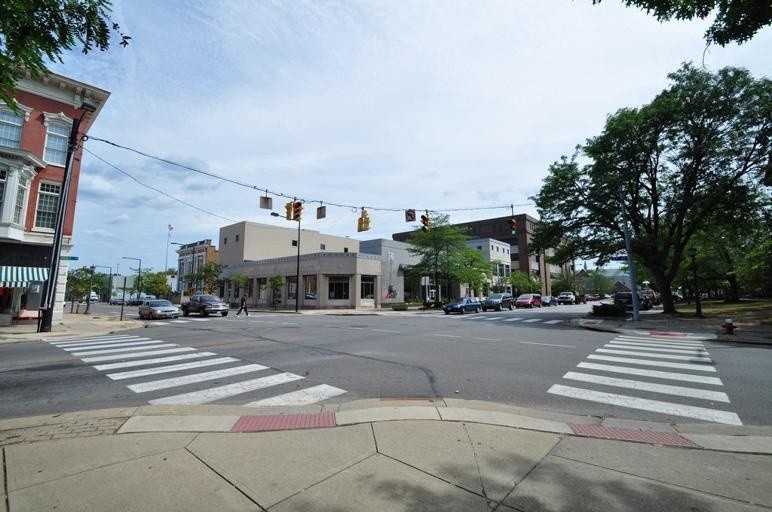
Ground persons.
[235,293,251,317]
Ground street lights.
[270,211,301,313]
[90,265,112,304]
[170,242,195,287]
[35,97,97,333]
[122,256,142,291]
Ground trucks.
[89,291,97,300]
[557,291,586,305]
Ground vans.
[482,292,541,311]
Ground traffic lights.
[508,217,517,238]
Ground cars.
[139,298,181,320]
[109,295,156,306]
[541,295,557,307]
[442,296,481,314]
[614,288,662,312]
[180,294,229,317]
